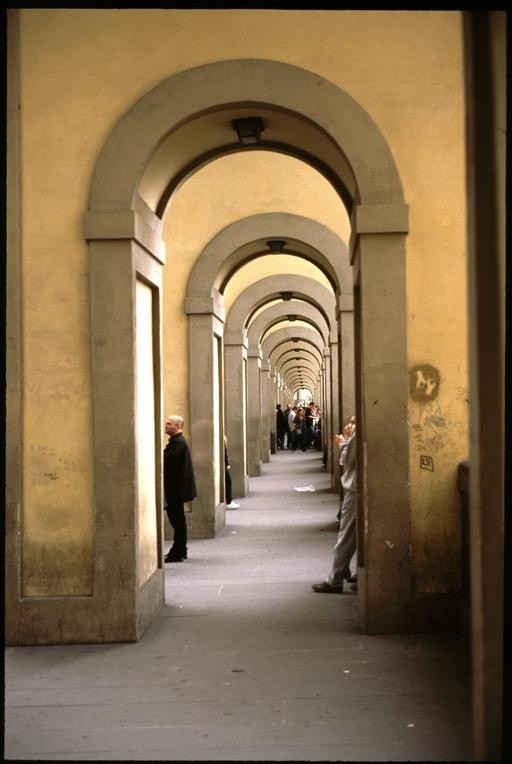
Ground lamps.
[279,290,297,322]
[226,115,266,148]
[265,238,288,254]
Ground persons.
[335,415,356,533]
[274,401,322,453]
[224,433,240,509]
[162,412,198,566]
[311,431,360,595]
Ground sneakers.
[226,500,240,509]
[164,550,187,562]
[346,573,358,593]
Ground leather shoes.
[312,582,344,594]
